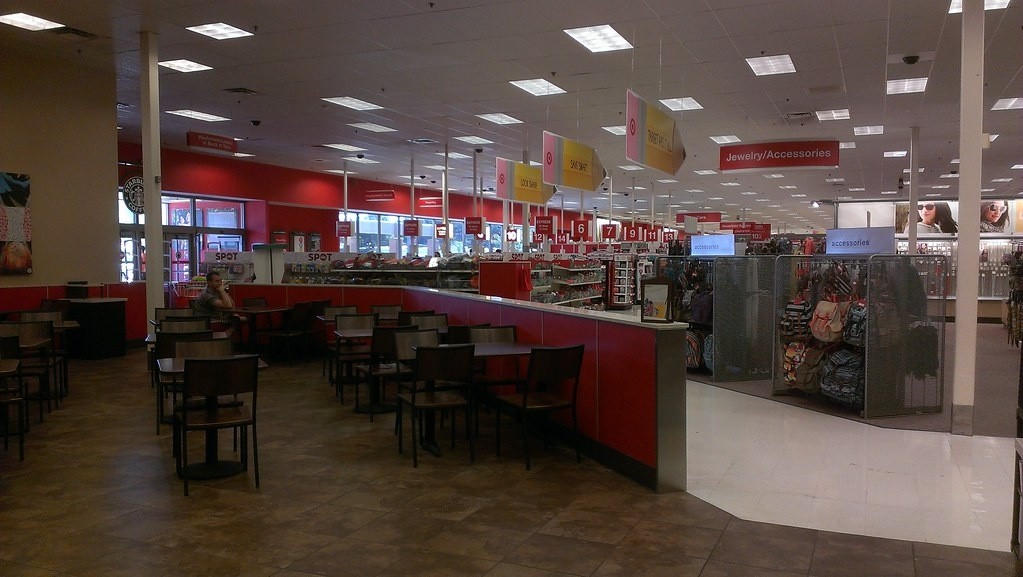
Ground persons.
[435,252,440,257]
[184,211,191,249]
[979,201,1010,232]
[916,242,928,254]
[177,215,187,250]
[140,246,146,280]
[981,249,988,258]
[904,202,958,235]
[193,271,246,339]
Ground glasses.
[917,204,936,210]
[987,203,1008,213]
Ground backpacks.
[781,291,865,409]
[681,290,713,373]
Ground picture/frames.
[639,277,673,323]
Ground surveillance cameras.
[737,218,740,220]
[356,155,365,158]
[475,148,483,153]
[593,207,597,209]
[950,170,958,174]
[638,218,641,220]
[249,121,261,126]
[654,221,657,223]
[603,188,608,190]
[903,57,920,66]
[624,194,629,196]
[633,199,637,202]
[431,181,436,183]
[489,188,493,191]
[420,175,426,179]
[483,190,487,191]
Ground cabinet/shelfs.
[205,241,671,313]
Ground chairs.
[354,324,420,423]
[156,330,214,437]
[172,337,246,474]
[370,303,403,323]
[278,299,332,363]
[410,313,449,332]
[396,344,476,468]
[393,328,461,450]
[322,304,360,384]
[151,308,195,388]
[467,325,527,445]
[0,310,65,461]
[440,321,491,430]
[160,319,208,399]
[174,353,260,497]
[335,314,377,404]
[166,315,211,330]
[494,343,587,470]
[397,310,436,326]
[257,301,312,368]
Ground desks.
[316,314,397,385]
[0,338,50,436]
[0,321,80,399]
[57,297,129,361]
[150,318,217,392]
[156,354,268,481]
[410,342,560,446]
[217,305,283,357]
[144,331,227,426]
[333,320,491,415]
[0,358,20,374]
